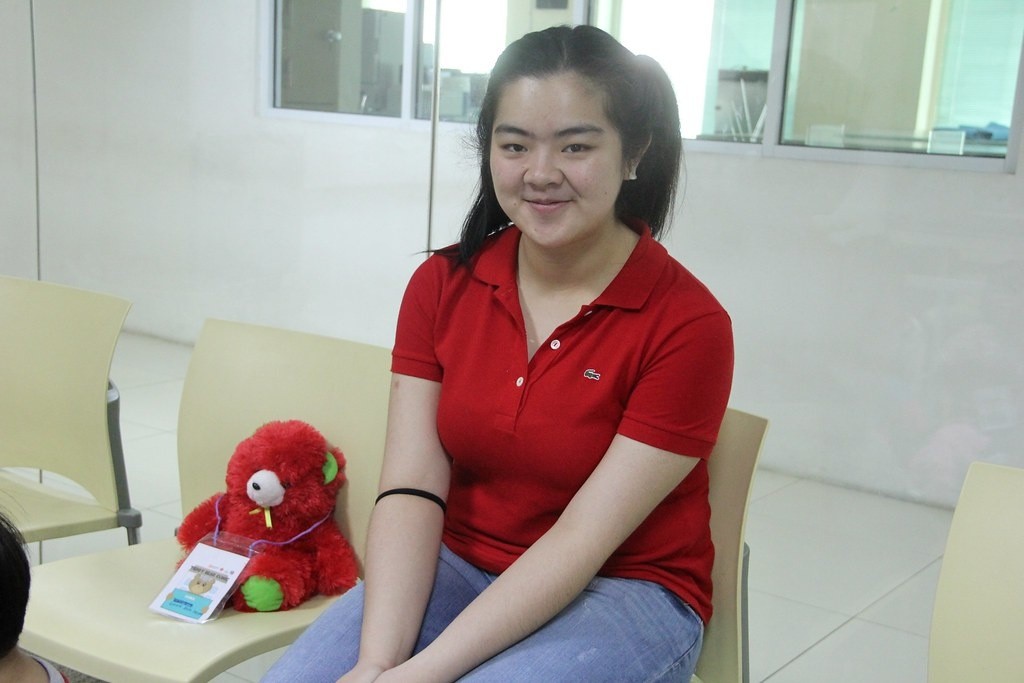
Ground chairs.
[0,272,143,547]
[688,407,769,683]
[13,319,392,683]
[925,464,1024,683]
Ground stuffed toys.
[177,421,359,613]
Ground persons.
[0,514,69,683]
[255,26,734,683]
